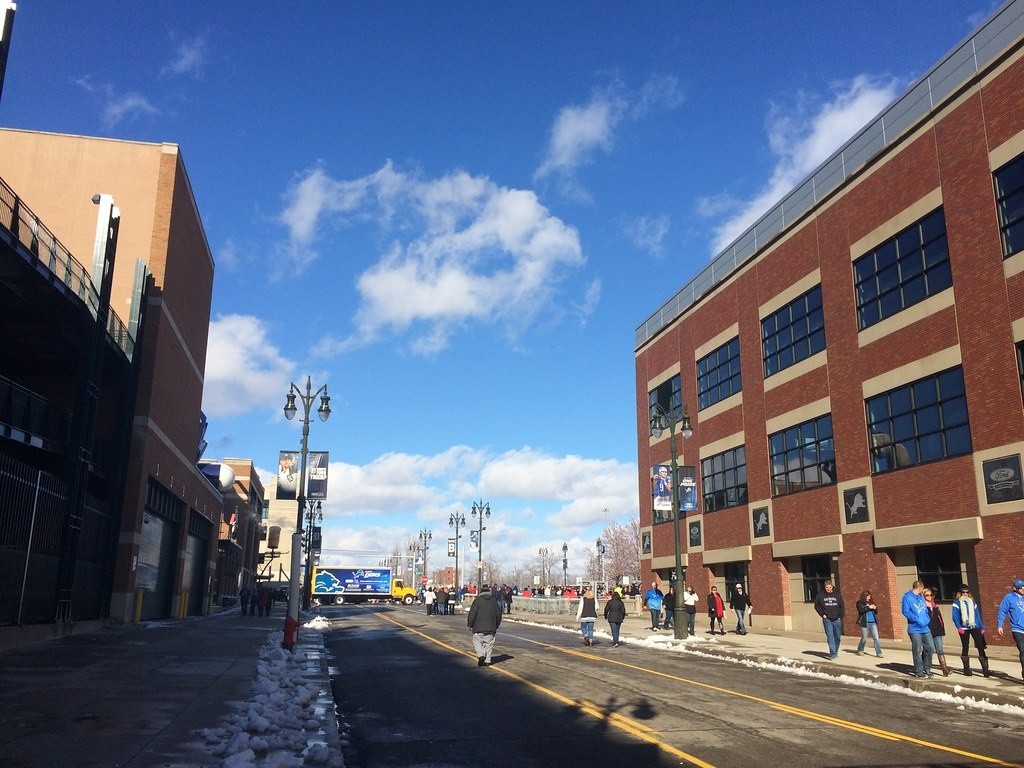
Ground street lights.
[595,537,602,581]
[393,552,402,579]
[538,547,549,585]
[651,394,694,640]
[449,510,467,594]
[408,541,421,591]
[561,542,569,586]
[419,528,433,603]
[282,374,333,651]
[305,507,324,609]
[302,497,323,611]
[470,497,491,594]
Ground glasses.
[925,594,932,597]
[713,590,717,591]
[962,591,969,594]
[661,471,667,473]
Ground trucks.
[309,565,420,607]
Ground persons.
[582,583,639,598]
[604,592,625,646]
[856,590,883,658]
[997,579,1024,678]
[654,467,671,501]
[278,454,296,492]
[902,581,952,677]
[458,584,518,614]
[730,584,753,635]
[643,581,699,635]
[707,586,726,635]
[576,590,599,646]
[468,584,501,667]
[952,584,994,677]
[814,580,844,660]
[423,587,457,615]
[522,585,579,596]
[239,585,277,617]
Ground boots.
[960,655,973,676]
[584,636,590,646]
[938,655,954,677]
[589,639,594,646]
[978,656,992,677]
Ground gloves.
[957,627,966,635]
[981,629,986,635]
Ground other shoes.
[858,650,864,656]
[923,666,933,675]
[478,656,484,667]
[484,661,494,666]
[613,644,619,647]
[653,628,657,632]
[913,671,926,678]
[876,654,884,658]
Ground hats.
[481,584,490,593]
[736,584,743,588]
[1013,579,1024,589]
[957,584,969,591]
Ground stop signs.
[421,575,428,585]
